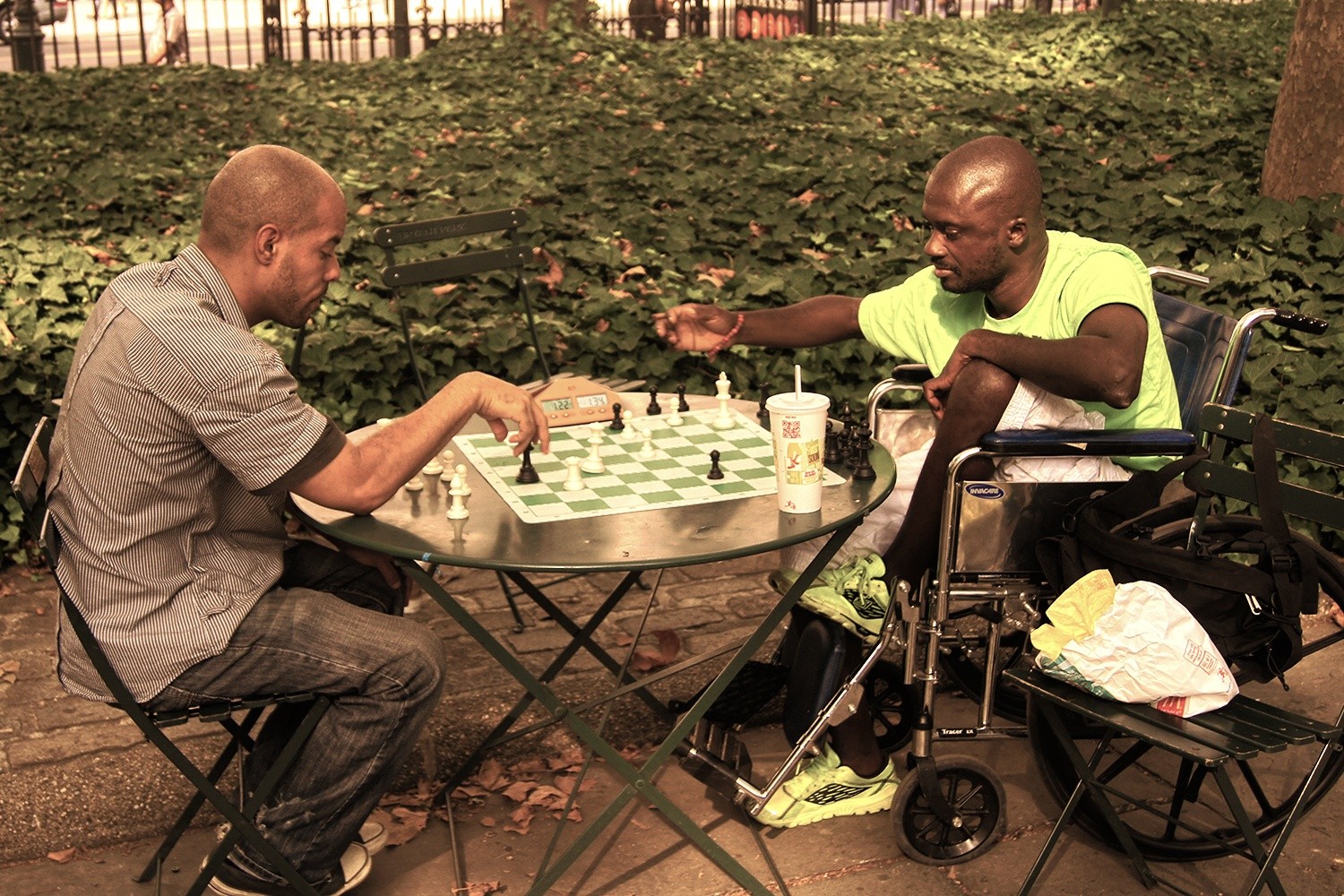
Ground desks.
[289,391,899,896]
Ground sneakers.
[766,551,890,648]
[746,741,898,827]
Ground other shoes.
[200,821,390,896]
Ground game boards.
[450,406,847,524]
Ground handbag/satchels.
[1072,469,1300,686]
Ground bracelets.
[706,313,744,357]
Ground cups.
[766,391,831,514]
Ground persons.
[655,137,1186,825]
[46,145,548,896]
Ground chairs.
[10,415,345,896]
[1001,402,1344,896]
[371,208,649,392]
